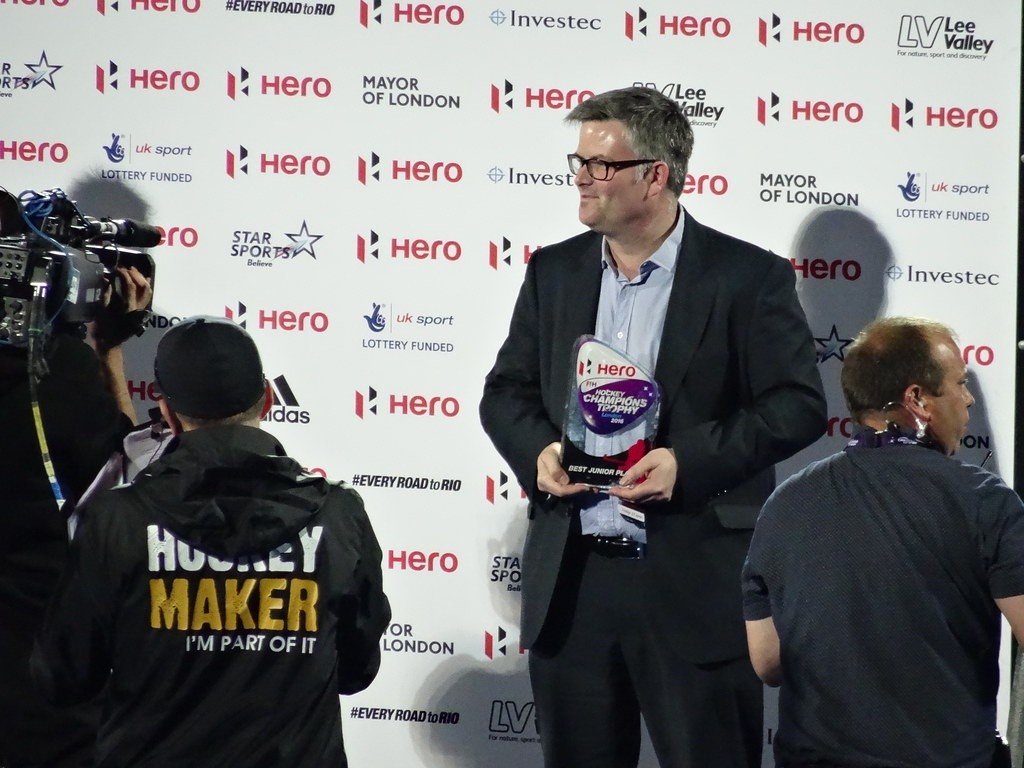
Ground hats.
[153,314,267,420]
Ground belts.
[579,536,646,559]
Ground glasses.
[567,154,661,181]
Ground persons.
[30,315,394,767]
[0,190,152,767]
[740,317,1024,768]
[475,85,832,768]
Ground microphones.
[90,219,162,247]
